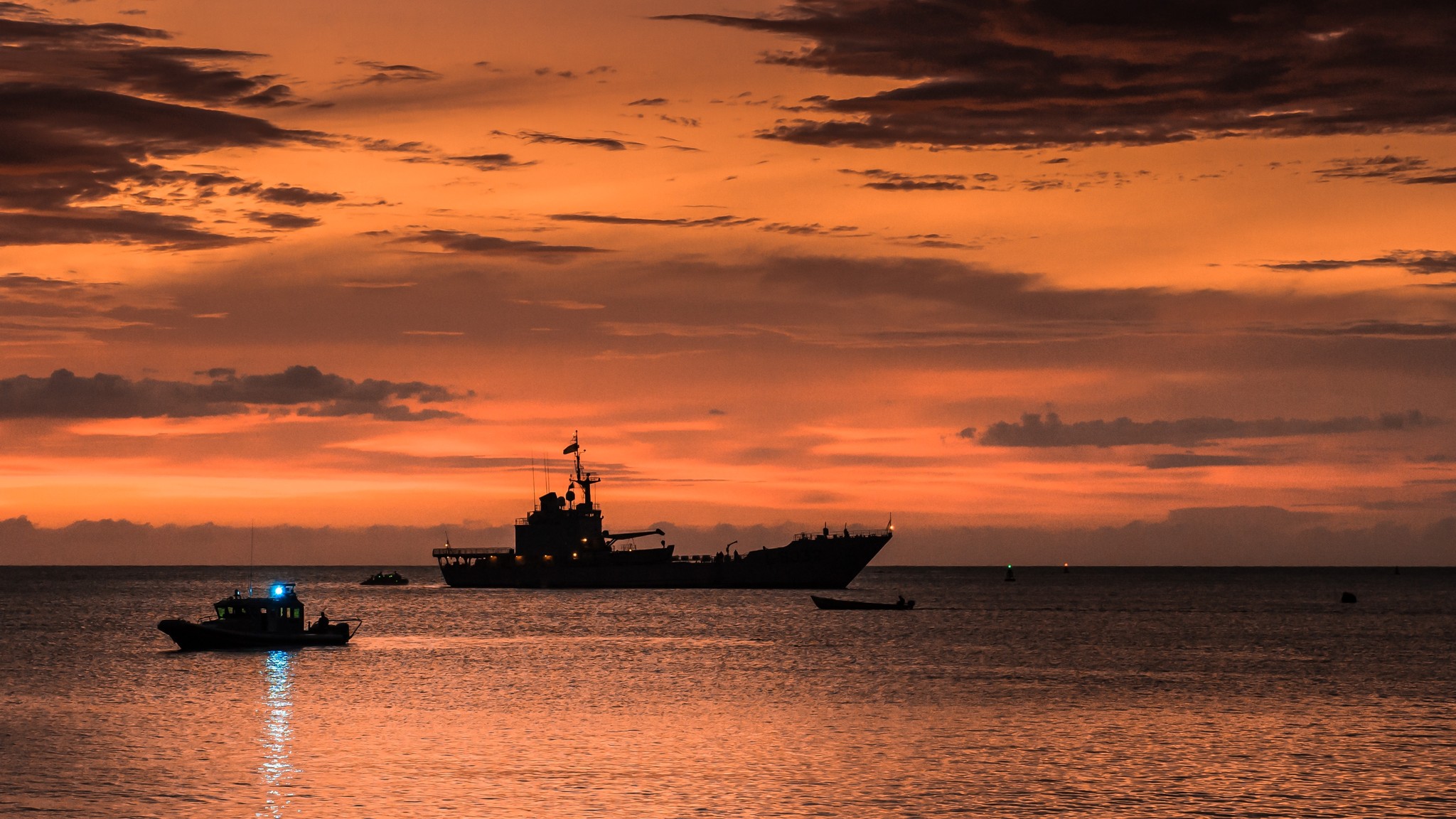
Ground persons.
[318,612,329,624]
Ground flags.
[563,443,578,455]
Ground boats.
[1004,565,1016,582]
[432,429,893,590]
[810,595,915,611]
[157,522,362,653]
[359,570,409,586]
